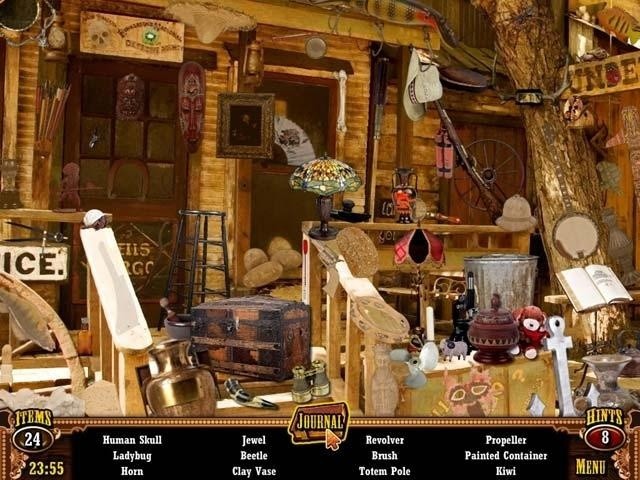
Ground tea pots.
[616,328,640,379]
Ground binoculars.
[292,360,330,404]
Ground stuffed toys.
[440,338,467,362]
[509,304,551,360]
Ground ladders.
[158,210,236,331]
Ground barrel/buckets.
[464,251,540,316]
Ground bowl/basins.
[164,312,192,339]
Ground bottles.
[291,365,312,404]
[310,359,332,397]
[145,337,218,419]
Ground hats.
[404,49,444,121]
[82,209,114,228]
[496,195,538,232]
[164,2,256,44]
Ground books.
[555,264,635,313]
